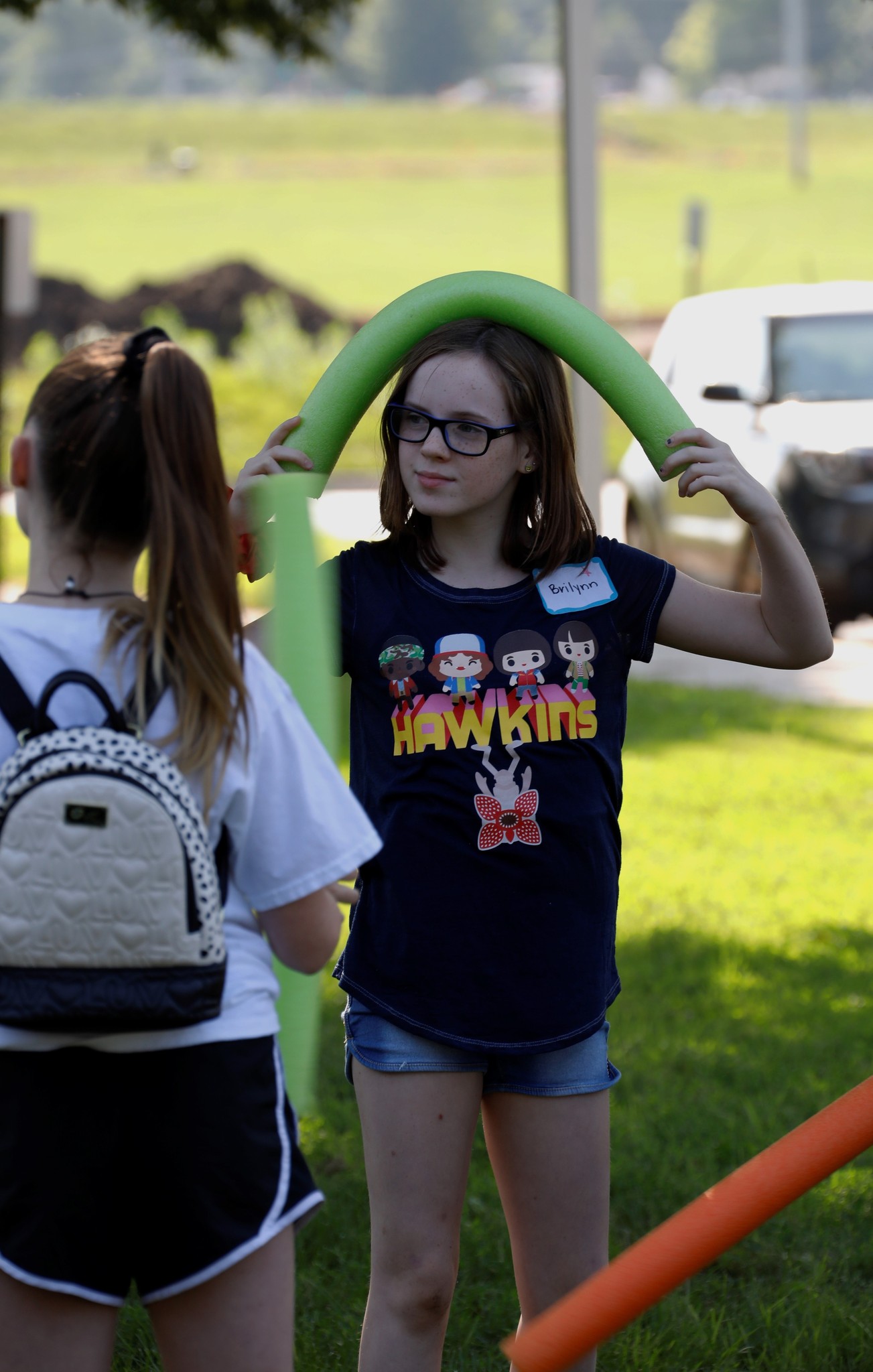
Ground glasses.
[384,402,519,459]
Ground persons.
[230,314,835,1372]
[0,321,387,1371]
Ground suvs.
[613,283,873,616]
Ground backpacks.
[0,655,231,1037]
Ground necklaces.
[18,575,137,601]
